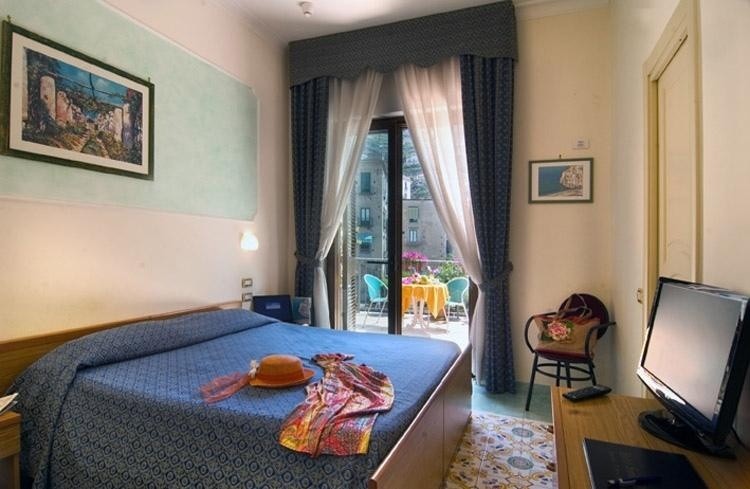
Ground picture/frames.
[528,157,594,204]
[0,18,156,182]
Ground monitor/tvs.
[635,277,750,462]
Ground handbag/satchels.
[533,293,603,361]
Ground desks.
[402,274,447,330]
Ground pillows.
[533,314,600,361]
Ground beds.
[0,301,472,489]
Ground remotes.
[562,384,612,403]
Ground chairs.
[524,293,617,411]
[445,277,470,326]
[362,273,388,329]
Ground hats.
[247,354,316,388]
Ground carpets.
[444,410,560,489]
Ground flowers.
[401,250,438,284]
[537,318,577,344]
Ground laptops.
[254,293,309,326]
